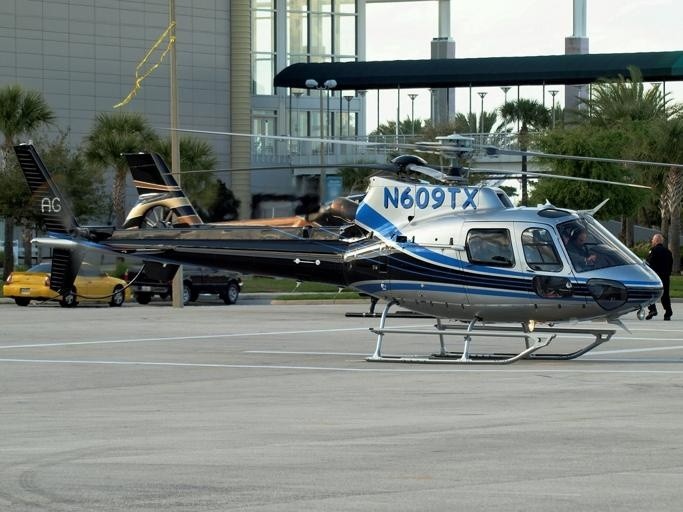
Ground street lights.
[479,92,486,145]
[409,94,418,145]
[430,89,439,129]
[652,83,662,113]
[575,85,583,111]
[292,92,303,154]
[305,77,337,205]
[344,91,366,140]
[548,90,559,127]
[502,88,511,149]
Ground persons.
[644,232,673,320]
[568,225,588,268]
[551,229,596,265]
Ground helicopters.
[14,129,683,365]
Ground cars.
[2,260,130,308]
[123,260,243,306]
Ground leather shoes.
[664,310,673,320]
[646,311,657,319]
